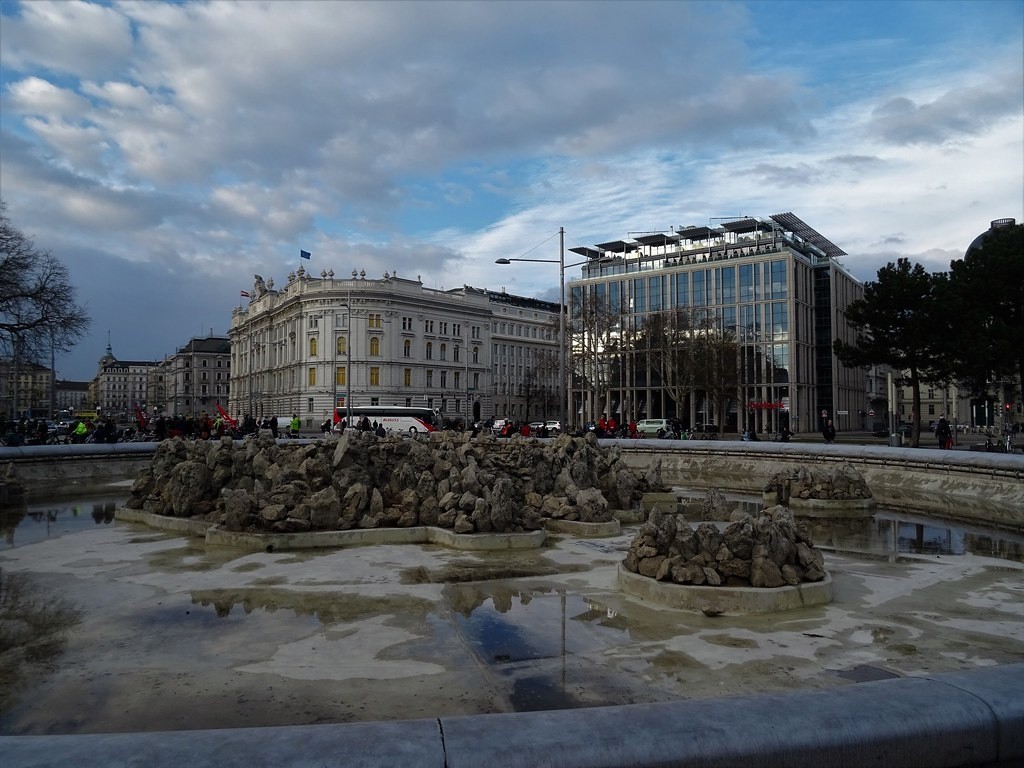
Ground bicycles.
[619,427,719,441]
[983,428,1016,454]
[0,420,297,445]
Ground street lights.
[237,321,275,420]
[143,338,195,418]
[341,289,392,428]
[495,224,615,434]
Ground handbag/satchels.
[946,438,954,448]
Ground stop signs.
[869,410,875,417]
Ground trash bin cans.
[889,433,902,447]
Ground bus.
[332,406,438,435]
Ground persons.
[584,414,643,439]
[823,420,836,444]
[657,417,682,440]
[321,417,386,438]
[2,410,278,445]
[469,416,549,438]
[289,413,300,438]
[936,414,952,450]
[780,427,794,442]
[740,427,757,441]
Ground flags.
[301,250,310,259]
[241,291,250,297]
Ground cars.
[474,418,562,434]
[873,423,914,438]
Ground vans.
[636,418,672,434]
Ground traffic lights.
[1003,383,1013,412]
